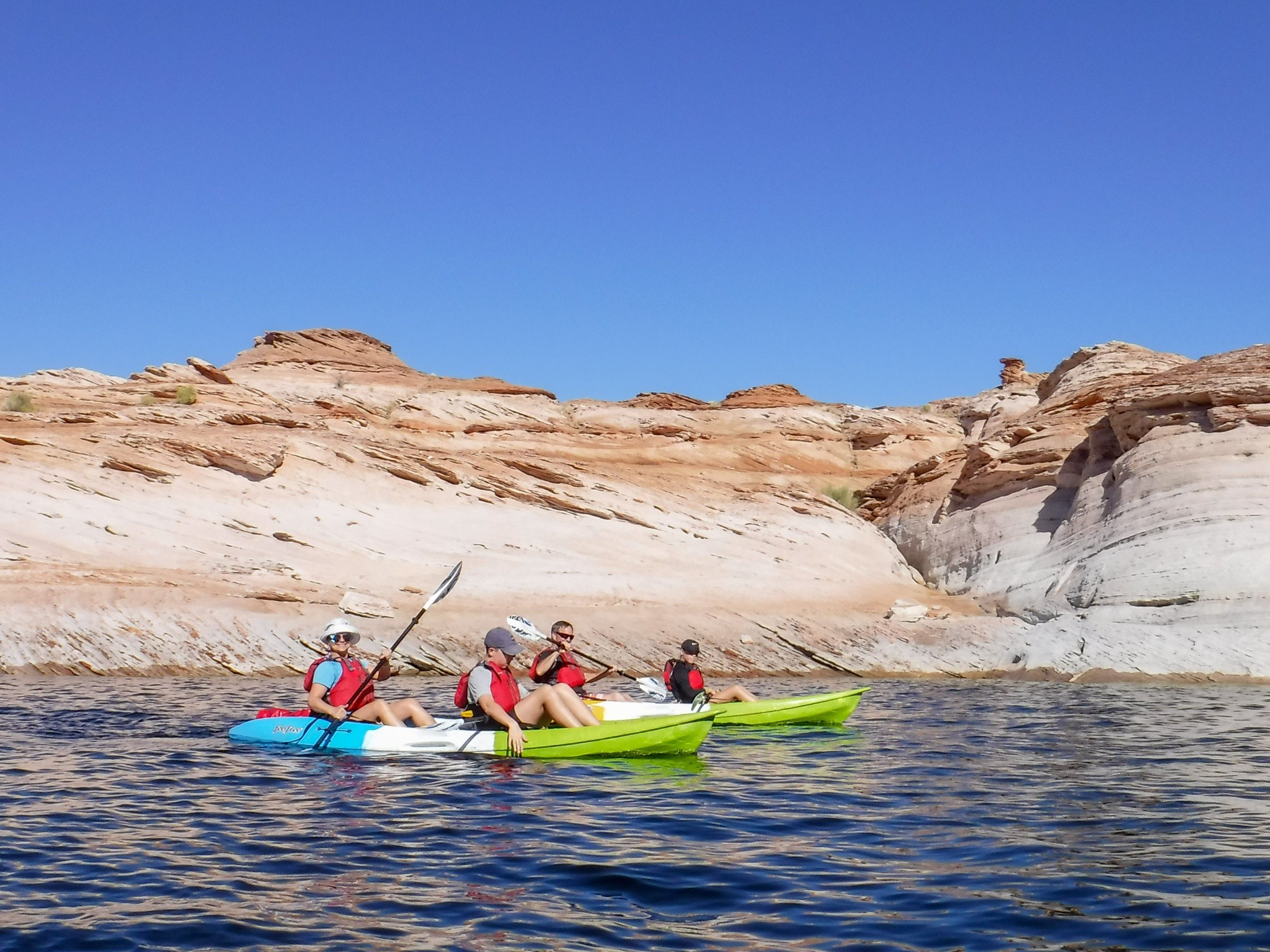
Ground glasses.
[554,631,575,641]
[328,634,354,643]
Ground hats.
[681,639,699,655]
[320,617,360,645]
[483,627,524,656]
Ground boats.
[224,705,728,760]
[548,683,873,728]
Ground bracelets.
[382,664,390,667]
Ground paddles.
[505,612,671,702]
[314,560,463,745]
[689,691,737,713]
[416,712,517,732]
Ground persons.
[663,639,759,704]
[454,627,601,754]
[303,618,437,728]
[528,621,638,702]
